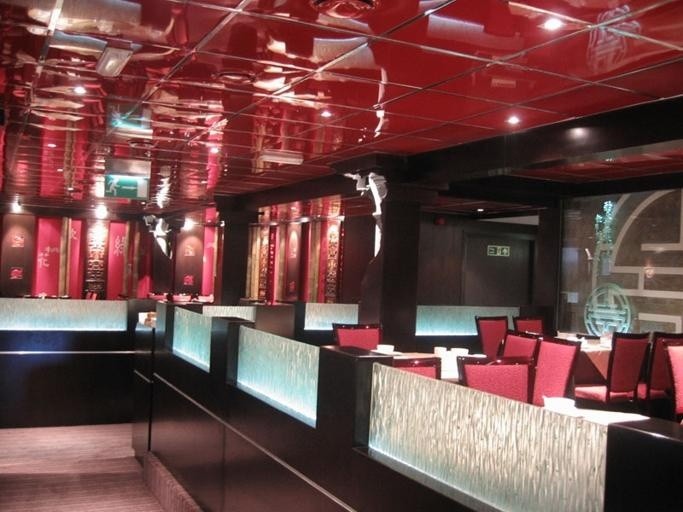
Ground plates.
[370,350,401,356]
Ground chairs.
[147,291,215,304]
[83,291,98,300]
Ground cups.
[451,347,469,356]
[376,344,394,353]
[544,396,576,413]
[434,347,447,354]
